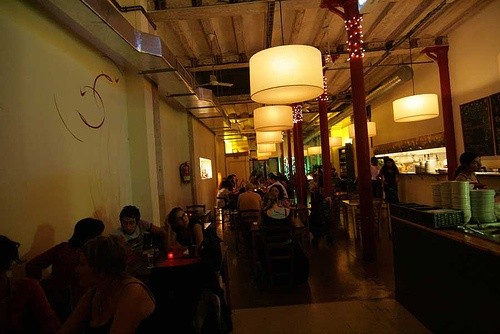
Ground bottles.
[148,243,154,267]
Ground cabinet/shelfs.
[338,143,355,179]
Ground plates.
[432,181,500,224]
[415,160,448,174]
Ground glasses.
[177,212,187,220]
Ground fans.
[196,34,234,87]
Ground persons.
[309,165,323,193]
[0,235,61,334]
[61,236,164,334]
[269,184,291,208]
[165,207,204,257]
[377,156,401,202]
[237,182,262,217]
[267,172,290,198]
[25,217,105,310]
[331,167,342,192]
[111,205,161,259]
[370,157,383,198]
[258,191,291,243]
[455,151,482,186]
[217,175,244,211]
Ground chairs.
[290,207,311,249]
[214,198,234,229]
[186,204,206,222]
[235,207,259,250]
[258,226,296,273]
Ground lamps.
[391,35,440,124]
[249,0,376,162]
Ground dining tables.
[126,256,202,270]
[342,200,392,240]
